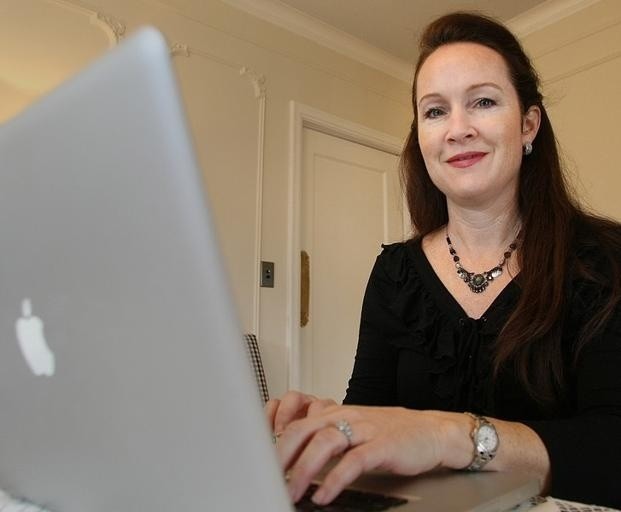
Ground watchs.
[462,412,499,472]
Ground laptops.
[0,24,541,512]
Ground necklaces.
[446,229,521,292]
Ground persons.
[263,10,620,508]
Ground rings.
[334,419,355,448]
